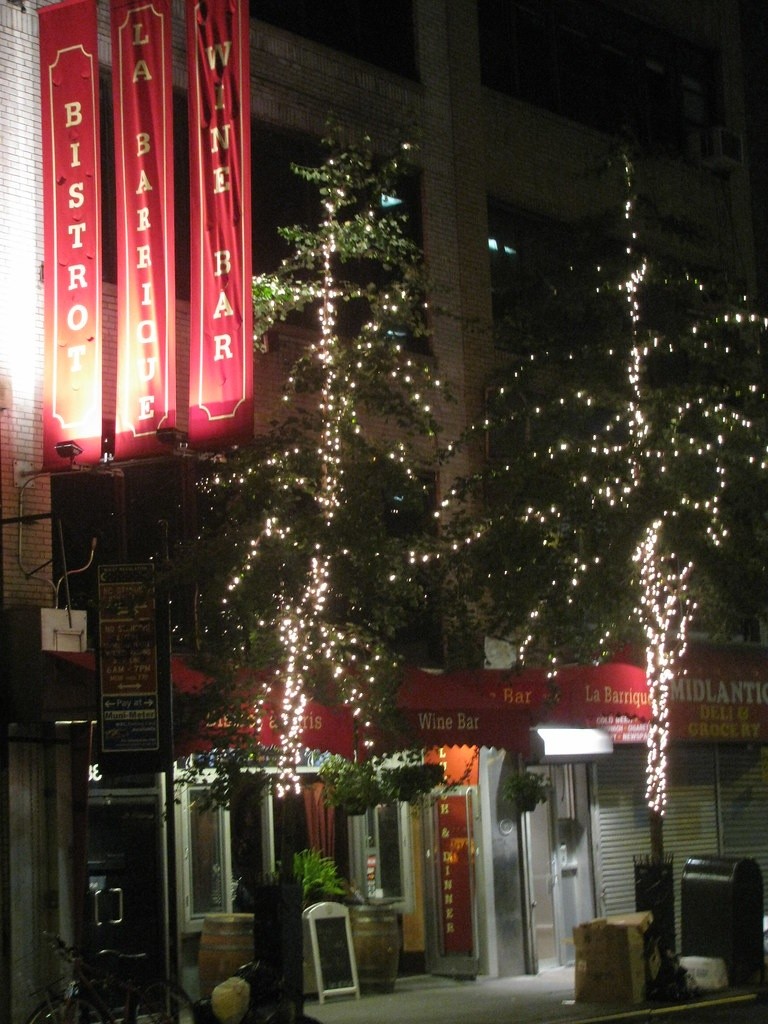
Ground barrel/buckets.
[350,906,399,984]
[199,914,254,998]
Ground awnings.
[437,642,768,751]
[60,648,531,759]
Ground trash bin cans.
[680,855,764,990]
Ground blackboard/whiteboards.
[308,916,359,998]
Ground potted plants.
[381,764,451,803]
[501,769,555,814]
[317,758,388,816]
